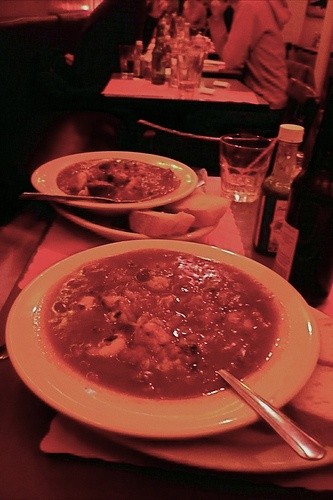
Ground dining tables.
[0,175,333,497]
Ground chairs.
[136,76,322,180]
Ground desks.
[100,72,273,154]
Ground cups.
[218,132,275,204]
[118,45,133,75]
[168,22,206,91]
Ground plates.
[54,207,218,241]
[116,302,333,472]
[5,238,320,439]
[31,150,199,214]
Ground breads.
[164,192,233,228]
[128,208,195,236]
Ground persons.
[65,0,288,176]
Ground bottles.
[252,123,304,257]
[151,24,166,86]
[269,107,333,310]
[133,40,145,78]
